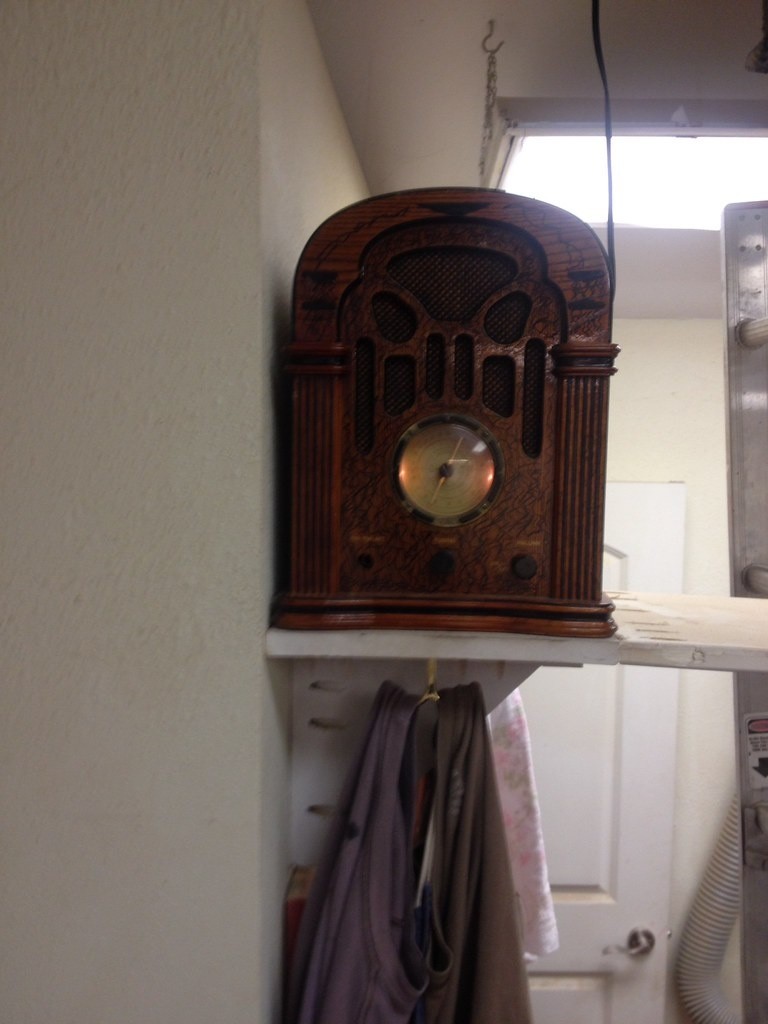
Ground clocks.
[274,189,621,638]
